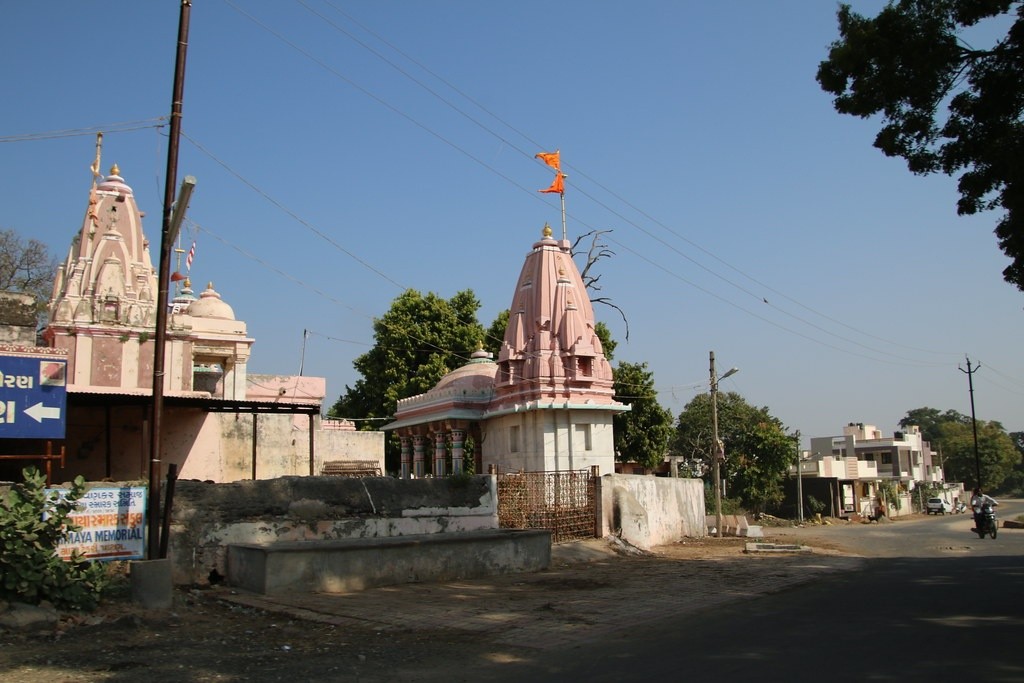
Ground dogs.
[867,514,880,523]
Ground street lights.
[710,366,740,538]
[795,454,813,522]
[939,456,950,499]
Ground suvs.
[926,497,954,516]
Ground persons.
[954,497,963,510]
[970,486,999,530]
[879,502,886,516]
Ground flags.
[186,240,196,271]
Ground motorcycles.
[970,499,1000,540]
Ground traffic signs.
[0,351,70,442]
